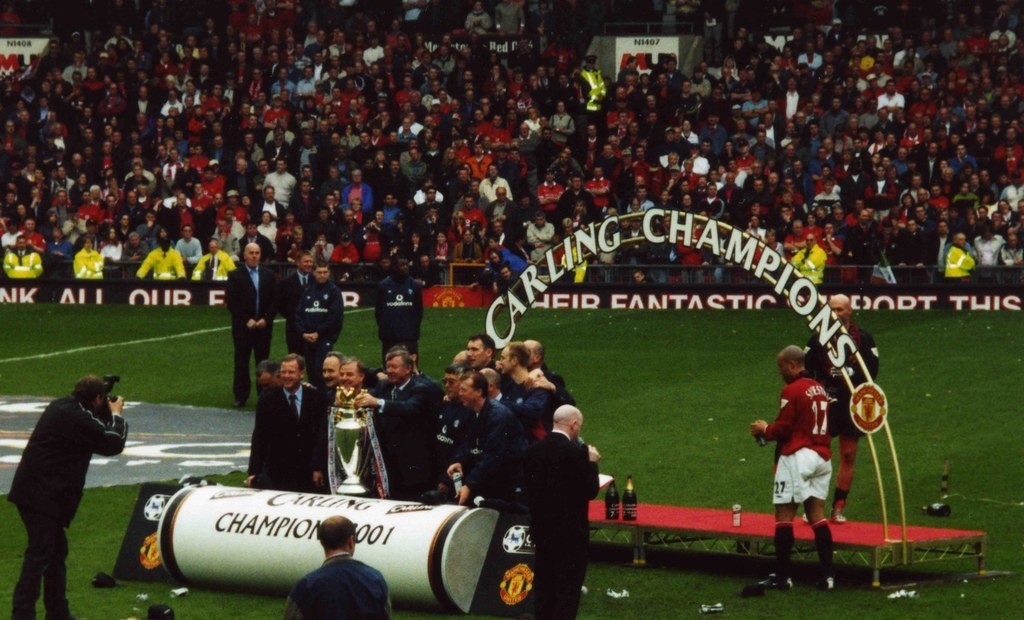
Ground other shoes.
[829,510,846,523]
[803,513,808,522]
[815,577,834,590]
[742,573,793,596]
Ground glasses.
[441,378,459,384]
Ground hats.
[209,159,218,166]
[227,190,239,197]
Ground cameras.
[95,375,121,421]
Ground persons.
[525,404,602,620]
[292,260,344,388]
[279,253,316,356]
[750,344,836,590]
[243,333,577,513]
[498,266,538,305]
[374,253,424,373]
[7,374,129,620]
[0,0,1024,281]
[224,243,279,407]
[283,517,392,620]
[147,604,174,620]
[802,293,880,523]
[469,248,538,293]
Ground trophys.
[325,385,377,496]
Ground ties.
[19,250,22,266]
[301,277,307,286]
[211,255,214,269]
[288,394,298,419]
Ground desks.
[589,500,988,592]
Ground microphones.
[576,436,588,450]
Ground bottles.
[605,475,619,520]
[622,475,637,520]
[922,502,952,517]
[731,504,741,527]
